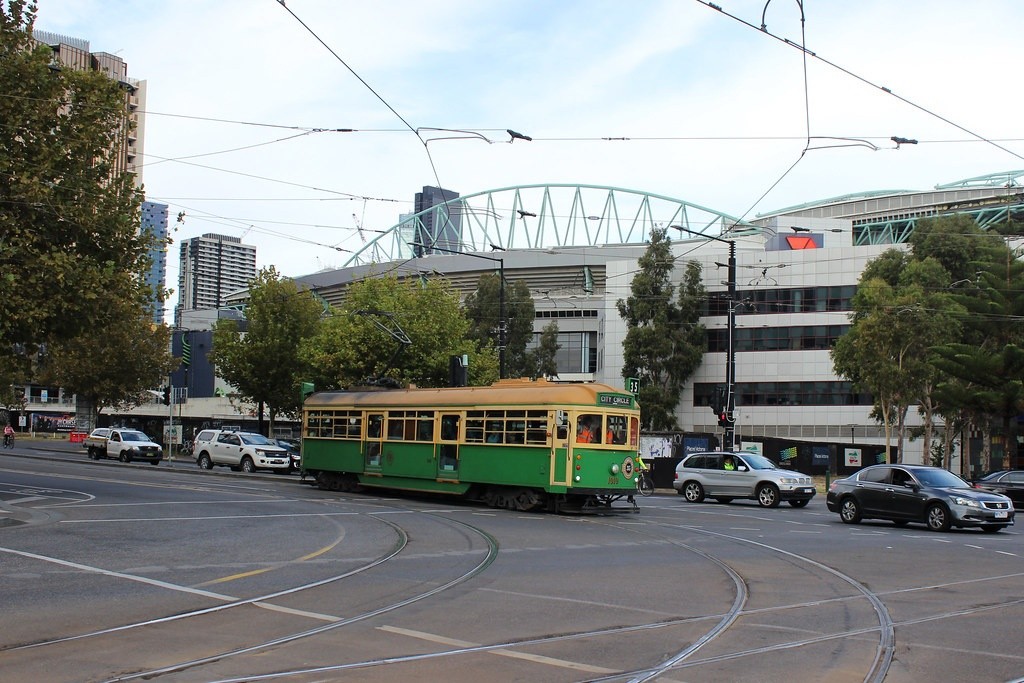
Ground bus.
[300,310,645,514]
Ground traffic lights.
[707,387,724,416]
[718,413,727,427]
[163,387,171,406]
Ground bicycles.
[637,468,655,497]
[3,432,15,449]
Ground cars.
[825,465,1016,532]
[268,438,302,474]
[970,470,1024,507]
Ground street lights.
[407,240,507,381]
[669,223,737,454]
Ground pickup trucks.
[82,427,163,466]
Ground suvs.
[191,428,291,472]
[672,452,817,508]
